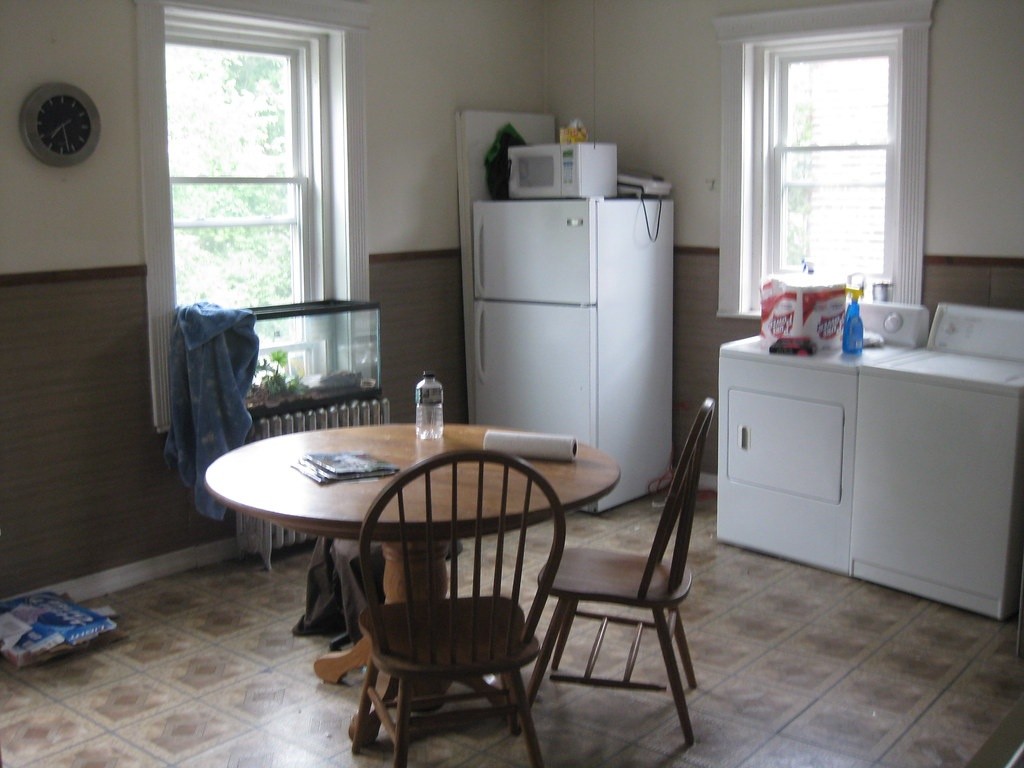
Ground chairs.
[347,451,566,768]
[507,396,717,748]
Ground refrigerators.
[470,196,675,513]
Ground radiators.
[225,394,393,572]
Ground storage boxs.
[244,300,385,421]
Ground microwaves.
[507,141,618,198]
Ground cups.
[872,284,892,302]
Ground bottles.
[842,303,864,355]
[414,371,444,439]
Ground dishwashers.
[716,300,930,574]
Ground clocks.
[17,82,101,165]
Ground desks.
[204,421,621,736]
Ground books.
[291,452,400,484]
